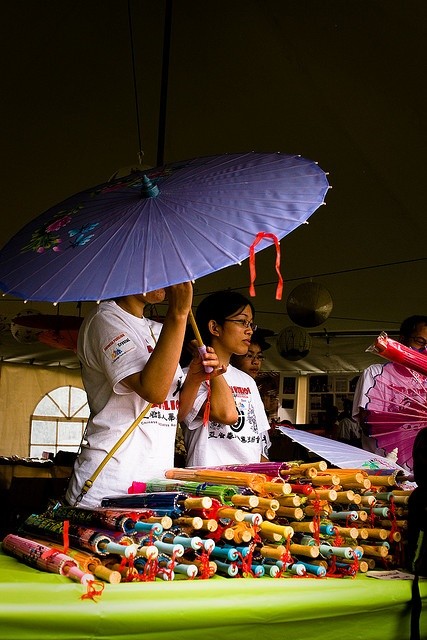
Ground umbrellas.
[375,336,427,374]
[275,425,419,491]
[330,466,416,492]
[364,362,427,471]
[25,302,84,351]
[127,459,329,492]
[0,150,333,374]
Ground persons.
[182,290,272,468]
[351,315,427,471]
[231,332,279,426]
[61,281,194,511]
[325,398,362,441]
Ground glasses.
[242,354,266,361]
[224,318,258,331]
[406,336,427,345]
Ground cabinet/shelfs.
[306,375,354,425]
